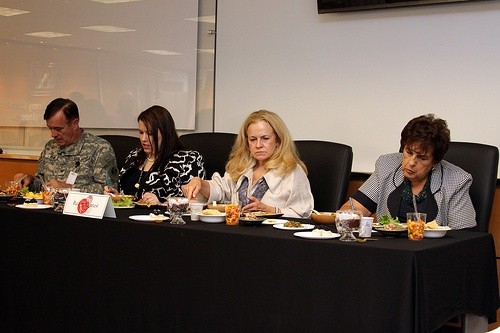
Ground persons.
[102,105,206,208]
[182,109,314,219]
[339,114,476,230]
[14,98,119,193]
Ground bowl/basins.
[195,213,226,223]
[423,228,453,238]
[207,203,225,211]
[35,198,43,204]
[376,228,408,235]
[311,212,336,223]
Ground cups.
[43,187,54,205]
[358,217,374,238]
[224,201,242,226]
[189,203,204,221]
[407,213,427,241]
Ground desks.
[0,200,500,333]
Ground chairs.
[292,140,353,212]
[97,134,141,171]
[399,142,499,232]
[178,132,238,181]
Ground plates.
[238,217,266,221]
[15,205,53,210]
[294,232,341,240]
[272,223,315,230]
[0,194,19,199]
[241,213,285,219]
[165,212,191,216]
[114,205,134,208]
[128,215,170,221]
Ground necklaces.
[133,159,156,189]
[146,156,158,162]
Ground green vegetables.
[377,214,400,225]
[22,187,30,193]
[111,195,137,206]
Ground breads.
[201,208,222,216]
[424,219,440,229]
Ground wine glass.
[335,210,363,242]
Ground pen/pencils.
[349,196,354,210]
[108,178,111,189]
